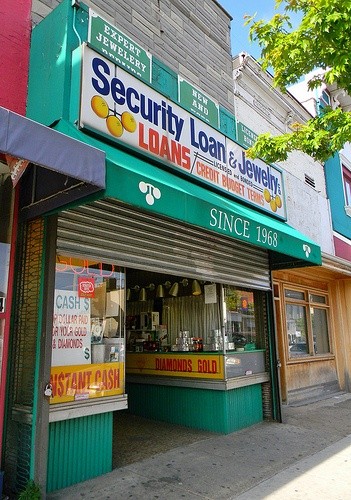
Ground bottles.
[152,343,156,352]
[143,342,148,352]
